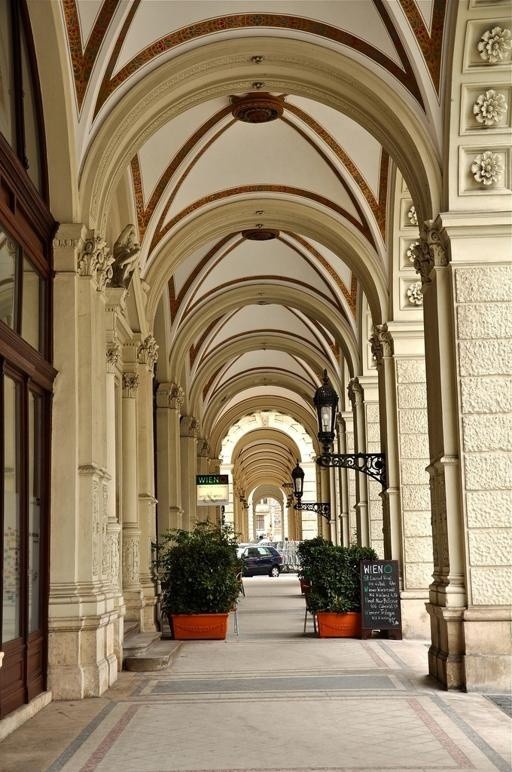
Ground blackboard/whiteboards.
[360,560,402,630]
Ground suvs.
[228,543,284,577]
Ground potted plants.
[151,518,246,640]
[295,537,379,638]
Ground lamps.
[313,368,386,488]
[292,459,330,520]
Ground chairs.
[303,585,317,634]
